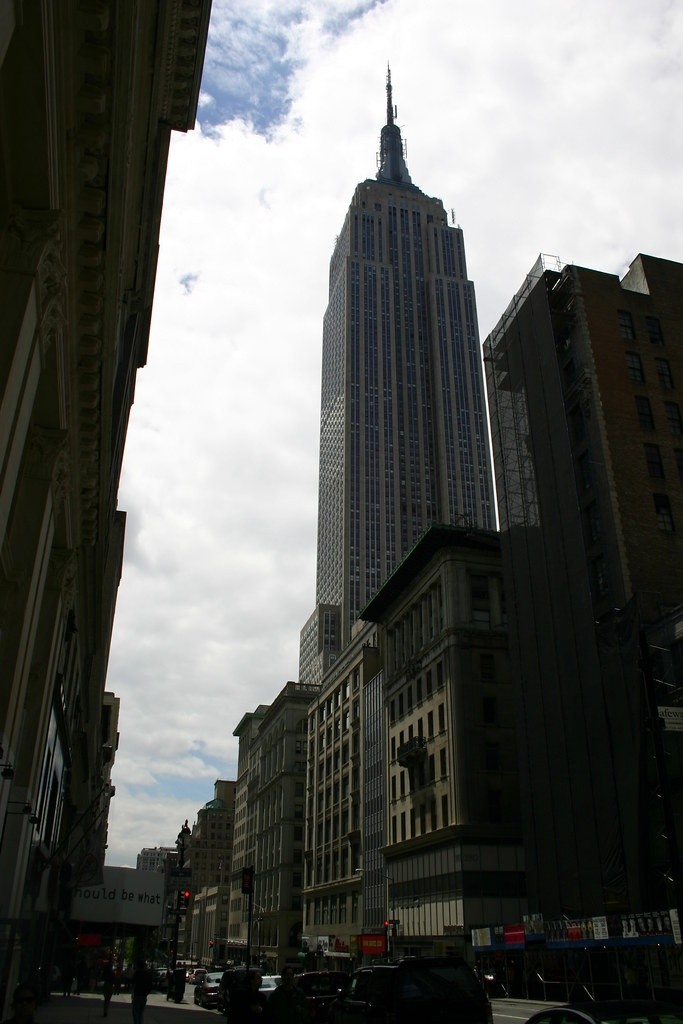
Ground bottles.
[580,921,594,938]
[546,924,568,941]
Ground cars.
[193,972,225,1007]
[525,997,683,1024]
[258,974,283,1007]
[185,969,208,985]
[261,970,349,1024]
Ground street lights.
[355,868,391,965]
[190,942,199,968]
[171,820,191,971]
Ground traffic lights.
[184,889,190,908]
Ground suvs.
[218,967,262,1013]
[326,952,494,1024]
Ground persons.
[114,975,120,995]
[63,982,72,999]
[123,960,174,1024]
[103,991,113,1017]
[228,967,312,1024]
[3,984,39,1024]
